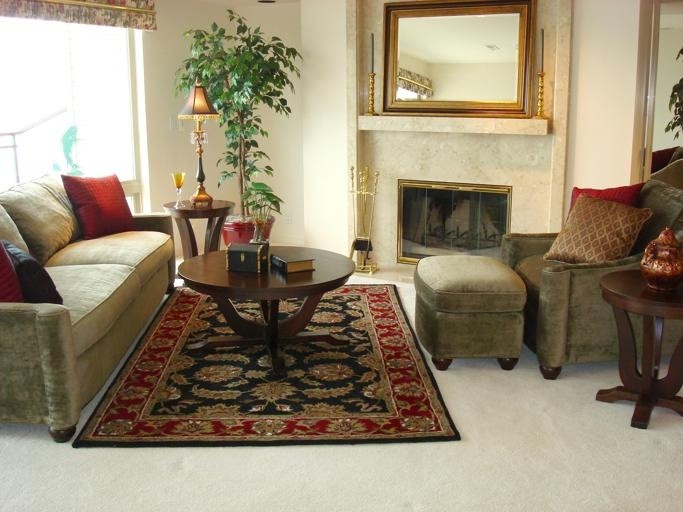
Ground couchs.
[0,178,177,438]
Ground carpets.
[70,283,464,449]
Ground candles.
[368,32,375,72]
[538,28,544,73]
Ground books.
[270,253,315,276]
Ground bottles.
[640,225,683,291]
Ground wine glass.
[171,172,187,209]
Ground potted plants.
[172,10,303,247]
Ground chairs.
[500,158,682,380]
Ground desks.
[178,244,360,371]
[595,269,683,428]
[163,199,235,264]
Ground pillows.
[59,173,140,239]
[0,241,25,303]
[1,238,62,304]
[542,192,654,265]
[0,182,81,267]
[0,203,28,254]
[567,181,646,222]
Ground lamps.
[176,77,220,204]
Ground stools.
[412,254,528,370]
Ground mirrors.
[380,0,537,119]
